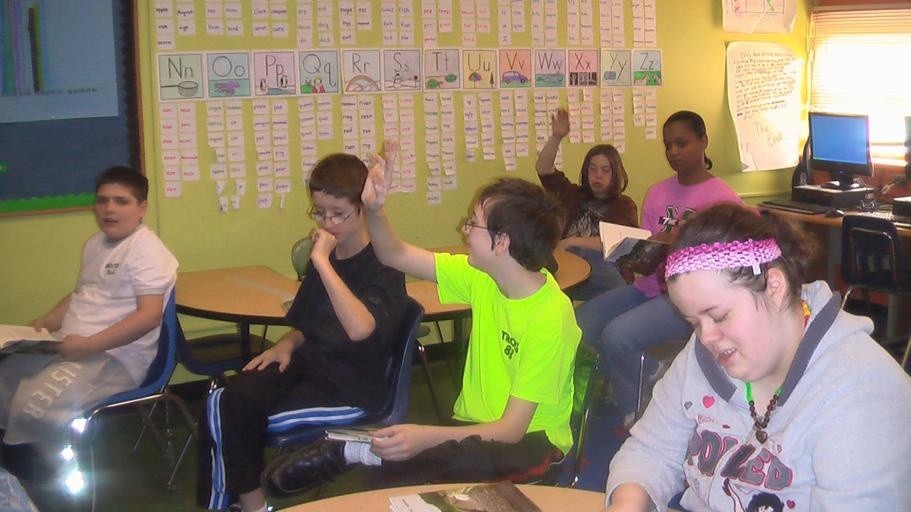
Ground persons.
[534,105,635,240]
[603,200,909,511]
[263,137,584,497]
[566,111,750,440]
[1,165,179,506]
[193,151,410,512]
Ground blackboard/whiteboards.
[0,0,146,218]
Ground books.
[321,420,395,445]
[1,322,64,356]
[598,219,671,276]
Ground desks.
[756,196,911,363]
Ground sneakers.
[259,435,357,500]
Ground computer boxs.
[791,185,875,209]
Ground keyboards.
[759,199,833,215]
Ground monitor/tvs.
[809,111,874,189]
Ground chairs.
[840,214,911,344]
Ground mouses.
[825,209,844,217]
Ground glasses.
[464,218,495,233]
[307,202,360,225]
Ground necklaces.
[744,299,812,445]
